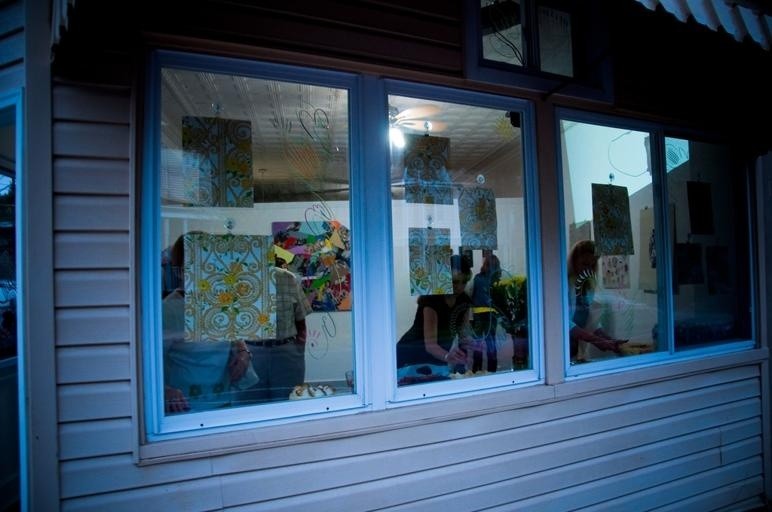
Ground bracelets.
[298,332,306,339]
[442,351,450,361]
[235,348,252,360]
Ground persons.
[397,255,486,370]
[160,231,253,415]
[567,240,629,361]
[235,266,313,405]
[276,257,289,271]
[470,254,502,373]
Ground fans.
[388,104,449,133]
